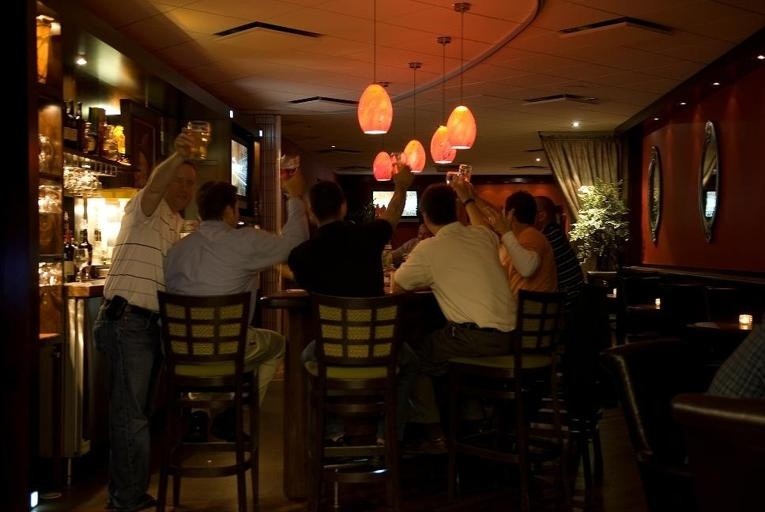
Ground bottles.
[64,232,76,284]
[76,230,91,282]
[380,245,396,284]
[64,98,126,165]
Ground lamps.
[357,2,476,181]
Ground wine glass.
[62,151,104,192]
[72,248,90,285]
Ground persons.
[158,172,308,448]
[91,131,199,512]
[388,173,584,463]
[703,315,765,402]
[288,165,413,467]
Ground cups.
[38,262,62,286]
[186,119,212,163]
[389,152,408,178]
[37,186,63,215]
[38,137,62,174]
[279,153,301,196]
[445,163,472,184]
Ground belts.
[102,300,160,321]
[448,327,484,337]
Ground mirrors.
[698,121,720,244]
[647,146,663,243]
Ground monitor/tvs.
[371,189,420,223]
[231,135,249,200]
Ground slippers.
[117,501,174,512]
